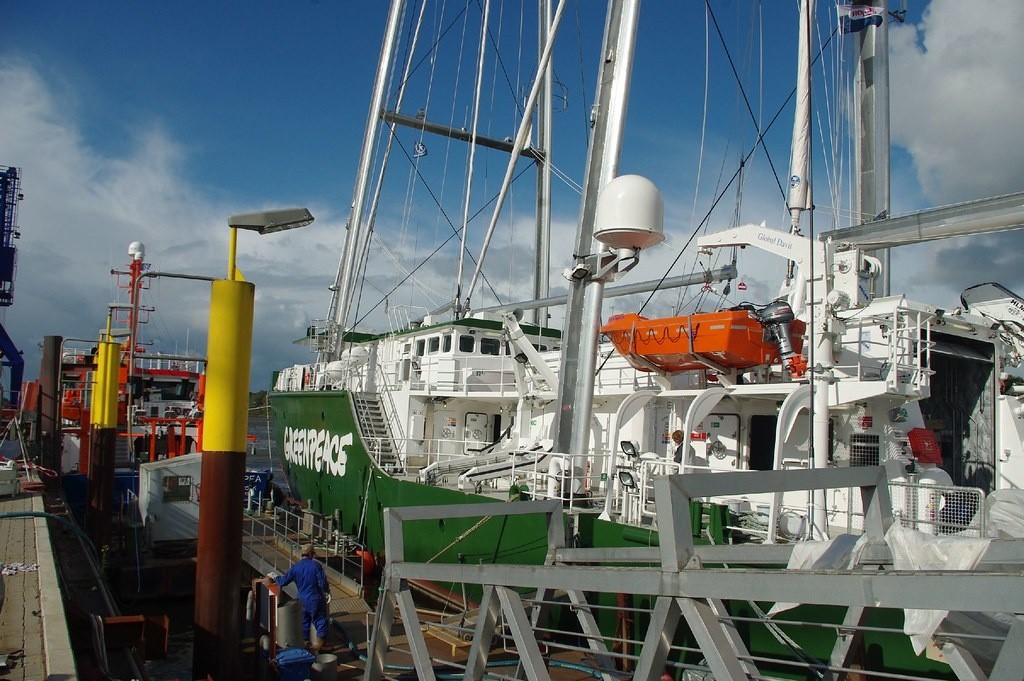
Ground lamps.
[619,470,639,488]
[562,267,576,281]
[514,353,527,364]
[620,440,640,458]
[413,361,422,370]
[572,263,593,280]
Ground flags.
[838,5,884,35]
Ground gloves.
[267,572,278,579]
[326,592,332,603]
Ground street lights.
[87,303,140,545]
[192,207,315,681]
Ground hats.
[302,543,316,555]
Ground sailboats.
[267,0,1024,676]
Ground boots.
[302,640,318,656]
[317,637,334,653]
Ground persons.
[268,545,333,652]
[672,430,695,465]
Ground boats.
[20,241,256,473]
[599,303,807,378]
